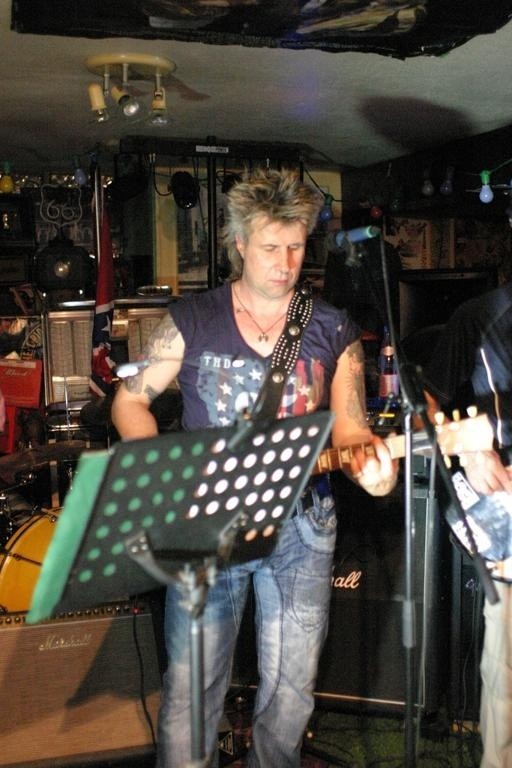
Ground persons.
[109,171,400,768]
[417,282,512,768]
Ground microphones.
[322,224,381,253]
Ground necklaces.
[233,282,287,342]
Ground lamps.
[166,166,247,211]
[0,159,14,193]
[68,142,90,189]
[86,53,178,128]
[319,165,496,221]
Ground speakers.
[230,425,436,706]
[0,601,162,768]
